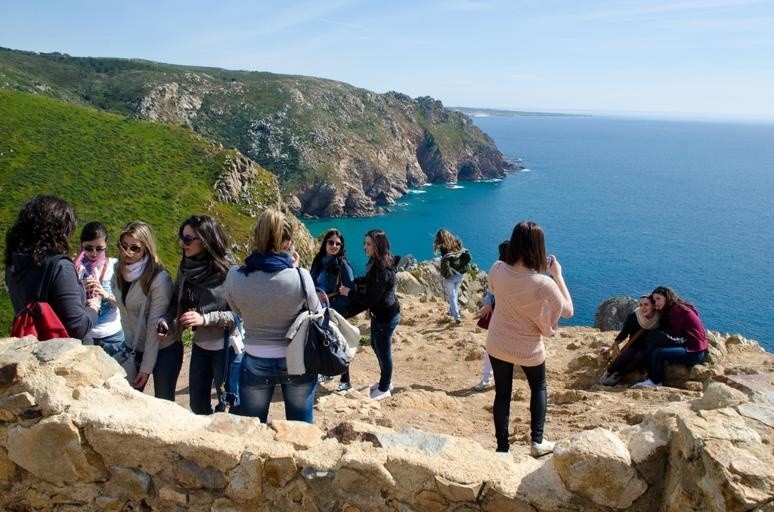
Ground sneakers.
[370,382,393,400]
[530,439,556,456]
[336,382,352,392]
[317,376,334,382]
[600,370,622,385]
[637,379,662,387]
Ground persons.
[631,285,709,390]
[5,195,102,345]
[218,207,317,423]
[110,218,185,406]
[472,241,512,393]
[482,220,577,459]
[69,221,124,356]
[220,297,246,411]
[431,226,469,326]
[154,213,237,416]
[600,294,662,388]
[335,230,403,400]
[309,228,356,393]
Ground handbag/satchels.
[303,317,350,377]
[10,300,69,339]
[650,329,688,345]
[221,351,244,408]
[121,353,137,387]
[477,304,494,328]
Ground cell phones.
[158,325,167,334]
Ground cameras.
[546,256,551,267]
[287,242,294,255]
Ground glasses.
[181,234,201,246]
[326,239,343,246]
[82,246,106,252]
[122,245,142,252]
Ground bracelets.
[88,302,101,311]
[106,291,113,303]
